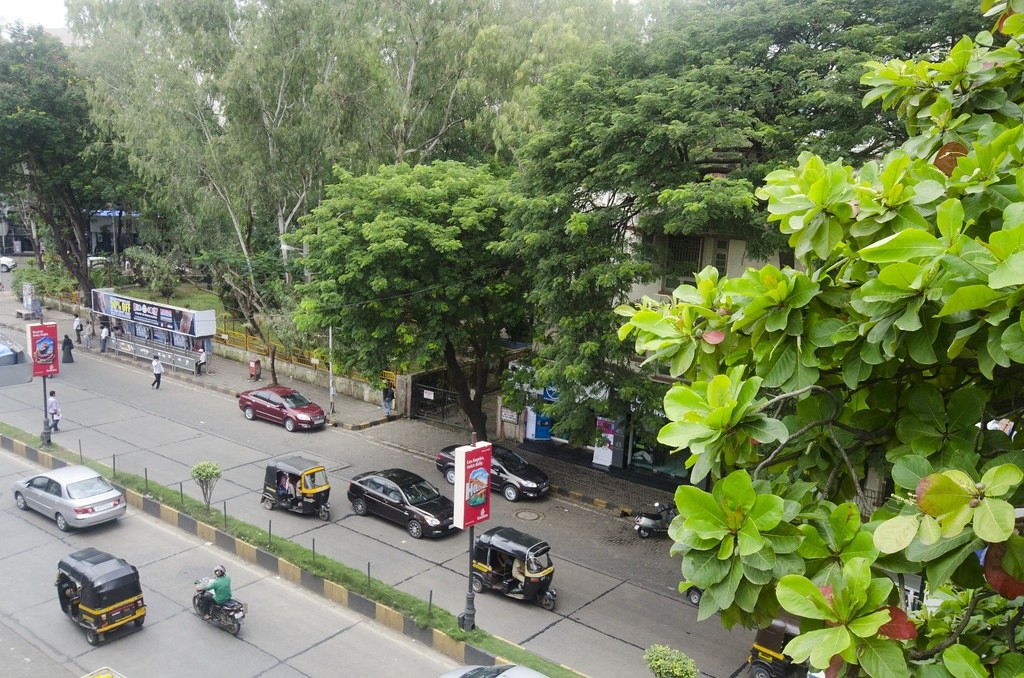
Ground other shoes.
[55,429,61,431]
[204,614,212,619]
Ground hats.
[199,349,204,353]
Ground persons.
[383,382,394,416]
[98,325,121,353]
[196,349,206,375]
[48,391,62,431]
[152,356,164,389]
[62,335,74,363]
[84,321,92,348]
[512,558,525,582]
[296,474,309,500]
[74,314,81,344]
[198,565,232,619]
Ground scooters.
[193,578,245,635]
[634,502,680,539]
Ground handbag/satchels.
[80,324,83,331]
[53,411,61,420]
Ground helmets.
[214,565,226,577]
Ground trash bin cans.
[250,358,261,381]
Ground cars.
[0,254,17,272]
[347,469,461,539]
[436,444,551,502]
[13,465,127,531]
[239,386,325,432]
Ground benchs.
[482,550,513,577]
[16,309,30,320]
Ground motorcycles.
[747,613,810,678]
[472,526,559,611]
[261,455,331,521]
[54,547,148,645]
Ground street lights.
[280,245,335,415]
